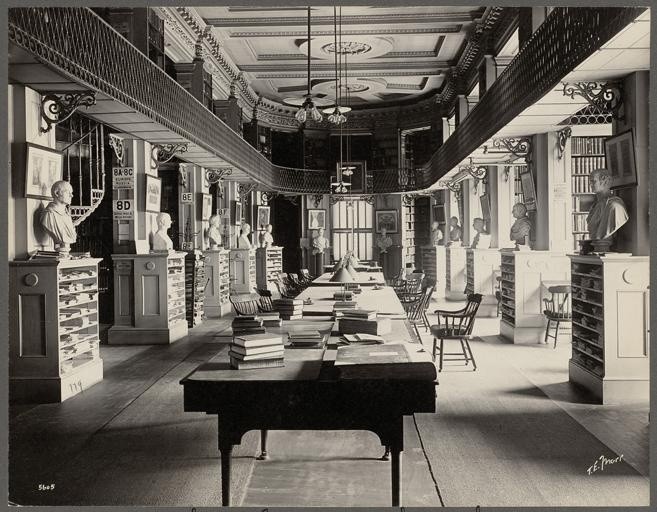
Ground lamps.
[281,5,357,210]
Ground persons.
[153,213,174,251]
[39,181,77,244]
[586,168,629,239]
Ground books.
[571,137,605,231]
[571,263,604,379]
[32,250,99,375]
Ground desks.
[177,316,437,507]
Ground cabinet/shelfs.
[403,198,429,276]
[571,134,612,256]
[9,245,284,403]
[106,7,274,169]
[419,241,650,409]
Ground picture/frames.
[234,201,242,227]
[306,209,326,231]
[602,128,639,191]
[23,141,65,201]
[374,209,398,235]
[519,170,536,213]
[200,193,213,222]
[256,204,270,231]
[478,193,491,223]
[432,203,446,225]
[143,174,162,213]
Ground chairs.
[224,257,434,372]
